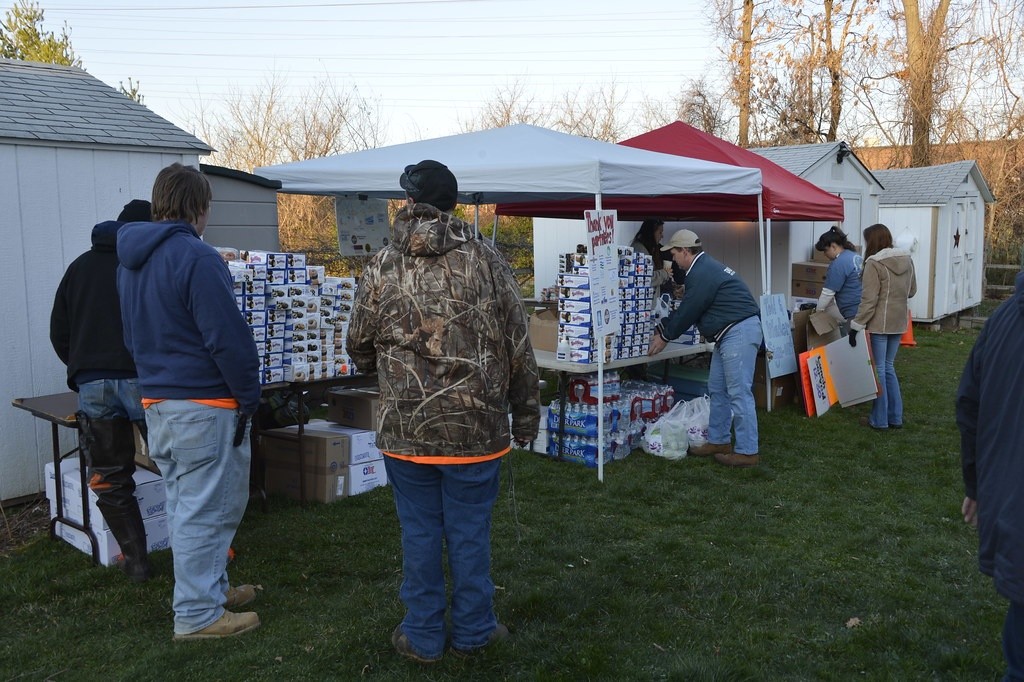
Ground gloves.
[848,329,857,347]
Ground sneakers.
[174,612,259,639]
[714,453,760,465]
[450,624,508,661]
[221,584,255,609]
[689,442,732,457]
[392,625,444,662]
[859,416,869,427]
[889,424,903,428]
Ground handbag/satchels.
[641,399,688,459]
[655,294,672,325]
[688,394,734,447]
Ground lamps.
[837,140,851,164]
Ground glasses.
[405,164,420,192]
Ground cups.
[663,260,672,272]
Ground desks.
[11,372,380,568]
[533,342,706,485]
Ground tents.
[492,122,845,410]
[252,123,767,483]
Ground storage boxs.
[507,405,550,456]
[775,377,796,410]
[751,368,778,410]
[528,245,655,364]
[792,245,863,311]
[214,246,363,386]
[669,300,705,345]
[259,386,392,505]
[43,423,172,568]
[637,365,711,406]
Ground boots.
[96,499,150,577]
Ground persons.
[114,163,260,638]
[649,229,764,467]
[815,226,864,337]
[849,224,917,430]
[955,271,1024,682]
[623,217,675,382]
[50,200,234,581]
[346,160,540,664]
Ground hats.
[660,229,702,252]
[116,200,152,222]
[400,159,458,211]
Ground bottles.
[548,371,676,467]
